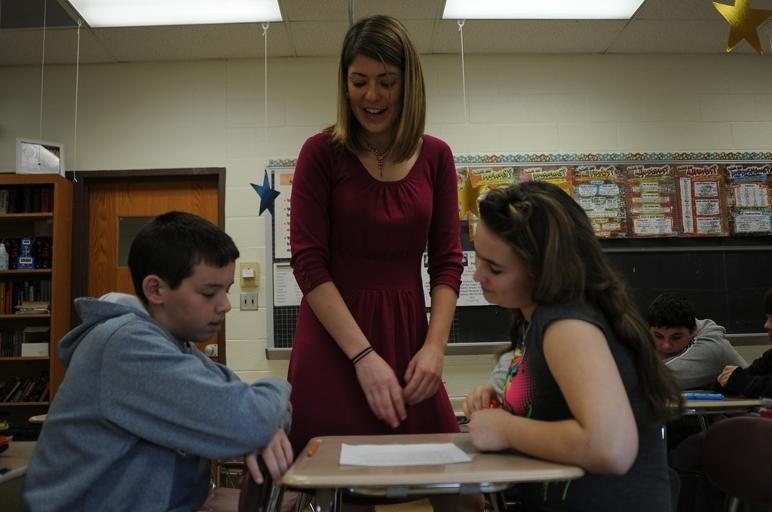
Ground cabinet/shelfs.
[0,174,73,442]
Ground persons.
[642,290,749,386]
[717,287,770,399]
[484,309,531,404]
[284,12,463,461]
[461,179,690,511]
[16,206,295,511]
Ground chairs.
[672,416,772,512]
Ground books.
[0,182,52,404]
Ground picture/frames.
[15,136,66,177]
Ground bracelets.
[349,345,375,364]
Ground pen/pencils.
[306,440,321,457]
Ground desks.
[280,433,585,512]
[663,387,763,435]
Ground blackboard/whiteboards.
[269,153,772,361]
